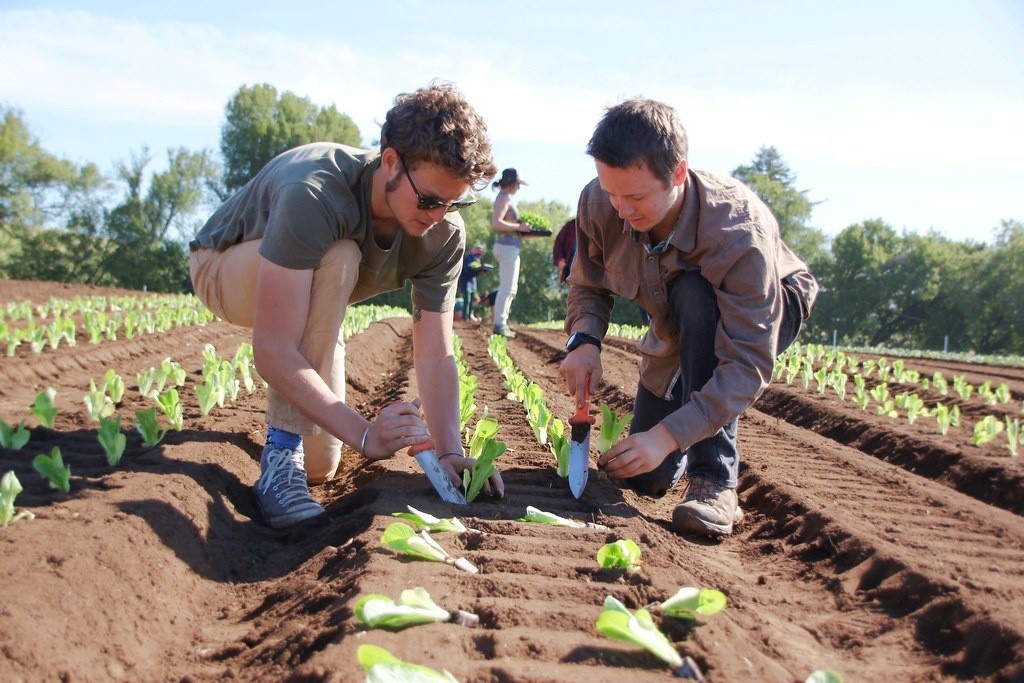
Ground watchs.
[565,332,601,355]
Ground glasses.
[399,153,476,212]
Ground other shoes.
[494,325,516,338]
[674,475,744,535]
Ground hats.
[501,168,528,186]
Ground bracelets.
[439,453,464,461]
[361,428,368,458]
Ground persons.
[558,98,820,536]
[553,220,579,284]
[492,167,531,340]
[187,79,504,530]
[457,244,487,321]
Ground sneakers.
[254,444,324,527]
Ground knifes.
[568,375,597,501]
[407,429,469,504]
